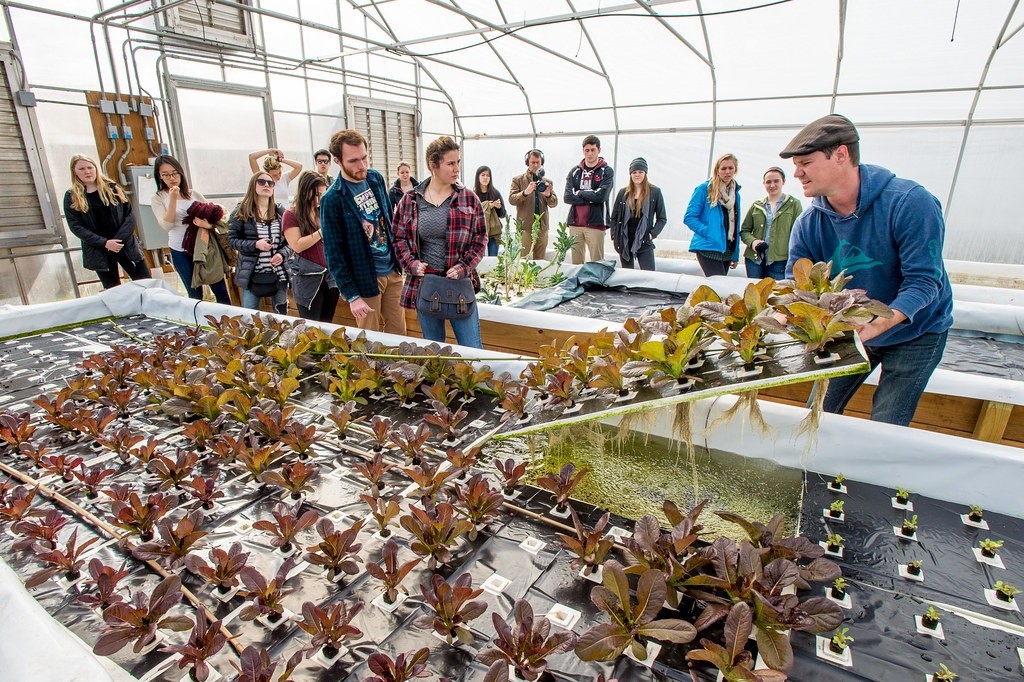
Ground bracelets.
[318,230,322,238]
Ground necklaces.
[428,186,451,208]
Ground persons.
[390,136,488,349]
[610,157,667,271]
[739,167,803,281]
[313,149,336,189]
[683,153,742,277]
[563,135,614,265]
[779,114,954,427]
[249,148,303,209]
[320,129,406,336]
[473,166,506,256]
[389,161,419,214]
[228,172,295,315]
[508,149,558,259]
[152,155,231,305]
[64,155,151,290]
[281,171,340,323]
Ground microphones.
[535,168,545,178]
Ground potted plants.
[994,581,1022,603]
[922,607,945,630]
[979,538,1004,558]
[906,560,924,576]
[932,663,961,682]
[901,515,918,537]
[0,256,895,682]
[969,504,984,523]
[895,484,912,505]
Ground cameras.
[755,242,769,254]
[535,180,546,192]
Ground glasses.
[256,179,276,187]
[315,159,331,164]
[162,173,180,180]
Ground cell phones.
[270,236,275,244]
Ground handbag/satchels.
[416,274,475,319]
[249,273,279,297]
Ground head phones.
[525,149,544,166]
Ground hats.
[780,114,859,159]
[630,157,648,174]
